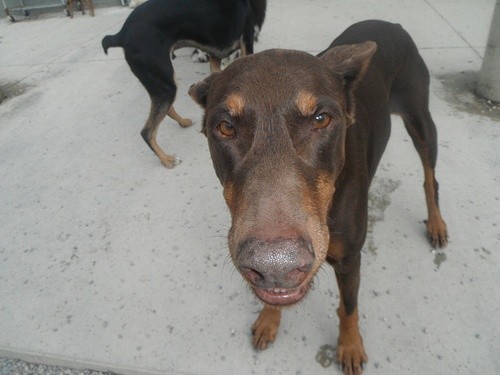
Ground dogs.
[188,19,450,375]
[101,0,266,170]
